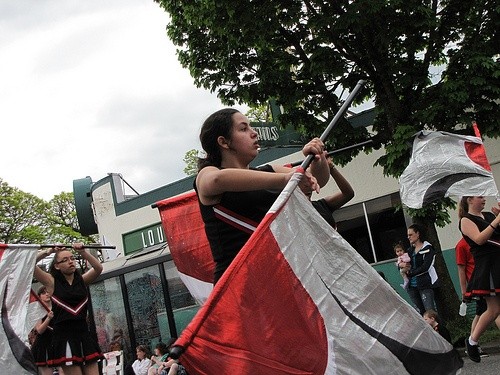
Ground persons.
[193,108,330,287]
[0,158,500,375]
[27,243,103,375]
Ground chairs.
[102,349,125,375]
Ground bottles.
[459,300,467,316]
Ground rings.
[406,267,407,269]
[49,312,52,314]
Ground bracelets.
[87,254,92,261]
[162,363,166,368]
[45,249,50,256]
[489,224,496,231]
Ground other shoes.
[465,338,481,363]
[479,350,488,358]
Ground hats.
[165,338,175,349]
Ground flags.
[172,169,465,375]
[154,163,293,308]
[397,124,500,209]
[0,244,41,375]
[26,289,50,335]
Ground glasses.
[57,256,76,264]
